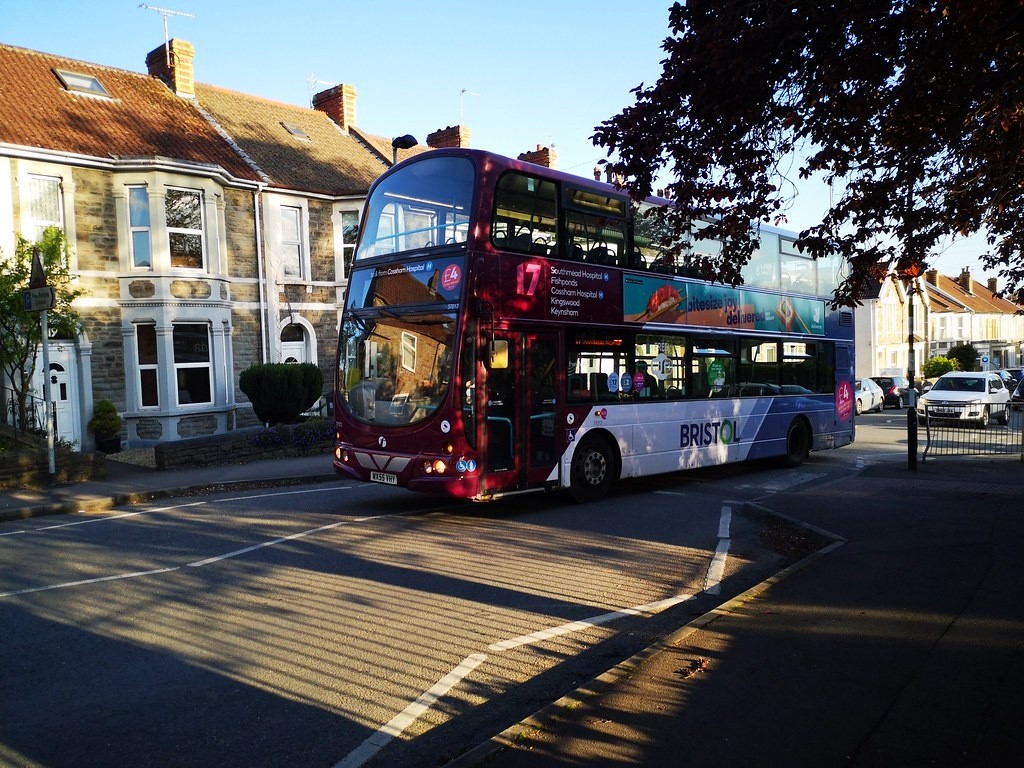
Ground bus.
[331,147,856,501]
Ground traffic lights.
[896,258,923,280]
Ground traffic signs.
[23,285,55,313]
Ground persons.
[714,371,725,392]
[438,334,455,394]
[916,377,932,395]
[635,361,657,395]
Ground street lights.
[392,135,419,250]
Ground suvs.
[917,371,1012,429]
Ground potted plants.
[86,399,121,454]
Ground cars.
[990,366,1024,411]
[868,375,919,410]
[855,377,885,417]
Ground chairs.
[669,385,767,399]
[425,218,814,292]
